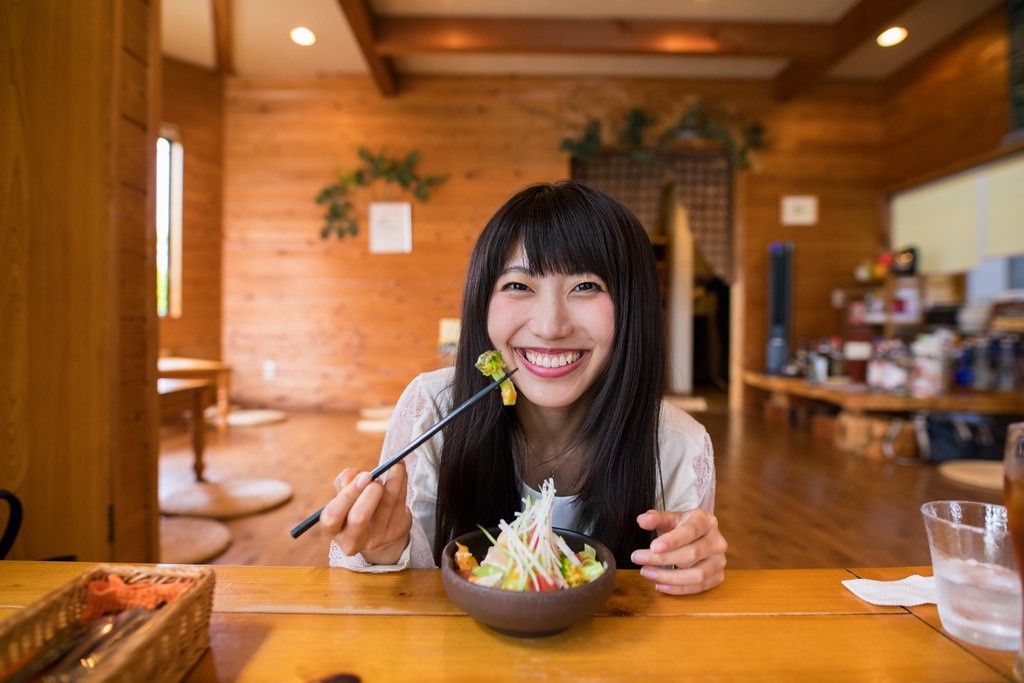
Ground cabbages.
[468,544,604,592]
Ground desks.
[158,379,216,481]
[158,357,234,431]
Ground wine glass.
[1004,423,1024,683]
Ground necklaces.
[522,435,587,482]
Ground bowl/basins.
[440,526,617,638]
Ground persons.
[320,179,727,595]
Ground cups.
[920,500,1024,651]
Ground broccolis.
[475,349,517,405]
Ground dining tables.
[0,561,1024,683]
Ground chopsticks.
[290,367,522,540]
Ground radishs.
[477,477,582,591]
